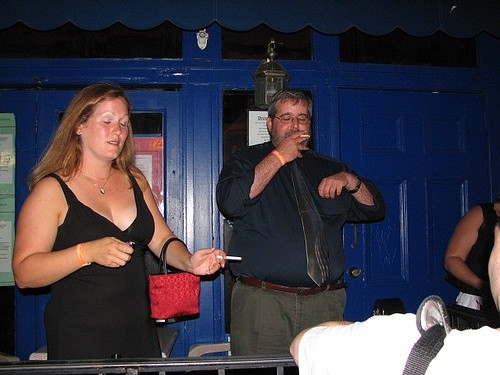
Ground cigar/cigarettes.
[216,256,242,260]
[300,135,310,138]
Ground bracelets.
[77,243,91,266]
[271,150,285,165]
[349,176,361,194]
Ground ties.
[289,160,333,287]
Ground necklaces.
[78,166,111,195]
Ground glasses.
[273,114,312,125]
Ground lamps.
[252,38,290,110]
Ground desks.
[0,352,297,375]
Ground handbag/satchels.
[148,236,201,319]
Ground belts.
[236,274,347,295]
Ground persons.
[444,202,500,328]
[289,220,500,375]
[217,88,385,356]
[14,82,226,375]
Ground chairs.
[372,298,406,316]
